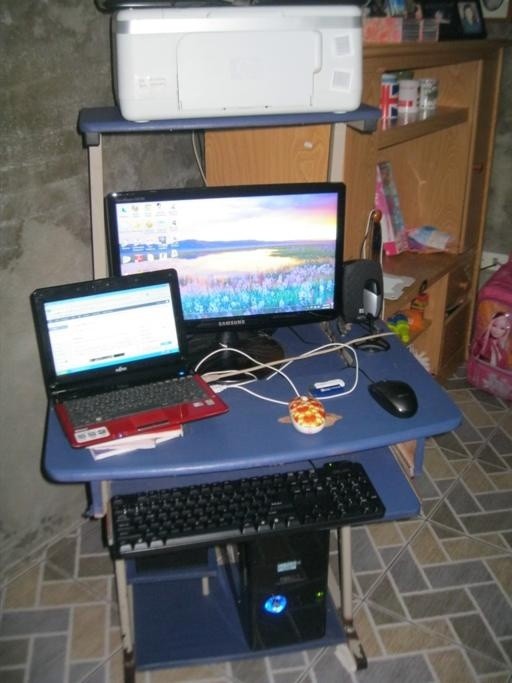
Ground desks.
[40,107,466,679]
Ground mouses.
[288,396,326,435]
[368,380,417,418]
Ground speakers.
[342,258,383,324]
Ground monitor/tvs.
[104,182,346,385]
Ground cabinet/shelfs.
[202,38,512,477]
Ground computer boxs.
[217,527,331,652]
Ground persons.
[473,300,511,371]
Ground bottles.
[395,312,411,344]
[381,74,399,120]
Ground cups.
[399,77,437,113]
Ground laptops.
[30,269,229,449]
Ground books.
[374,158,408,256]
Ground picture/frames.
[453,0,512,40]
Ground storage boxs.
[114,7,369,128]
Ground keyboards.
[110,460,386,555]
